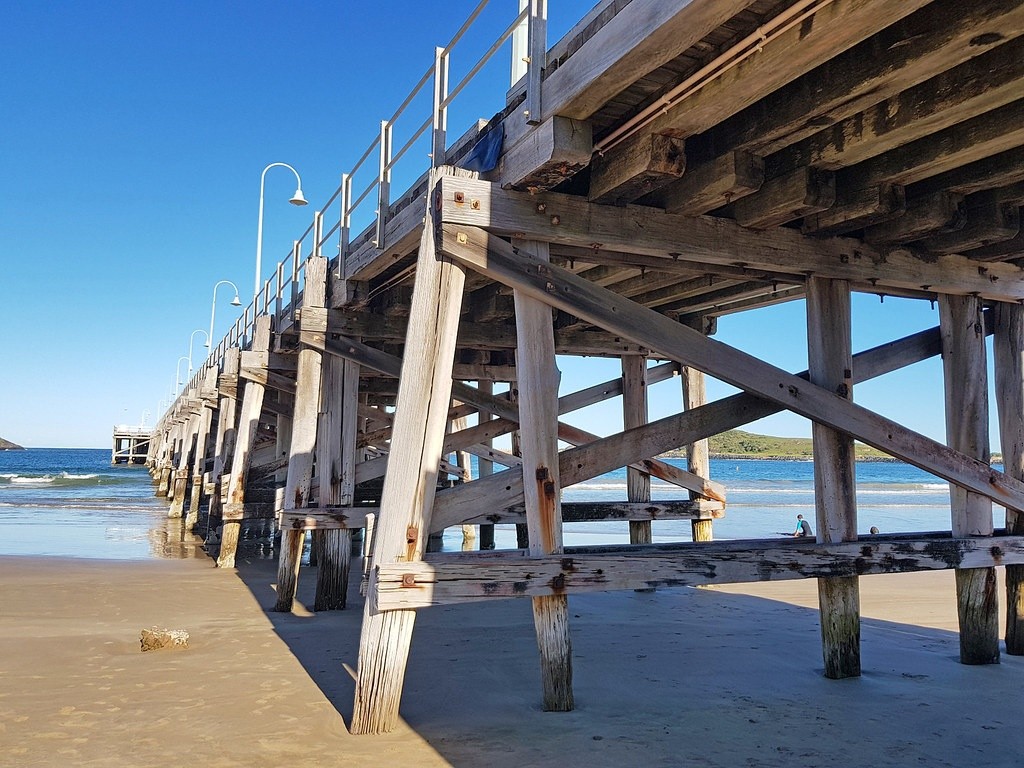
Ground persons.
[794,514,813,537]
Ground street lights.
[207,281,242,357]
[187,328,211,384]
[175,356,193,397]
[252,160,310,328]
